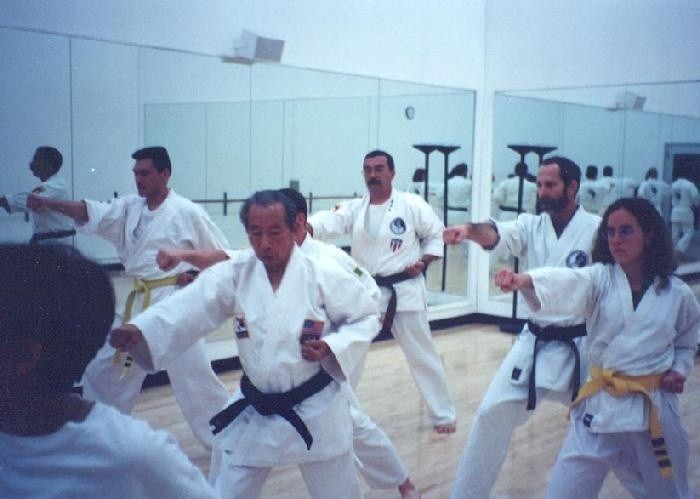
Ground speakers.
[238,28,285,63]
[624,91,647,111]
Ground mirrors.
[0,26,478,342]
[489,77,700,306]
